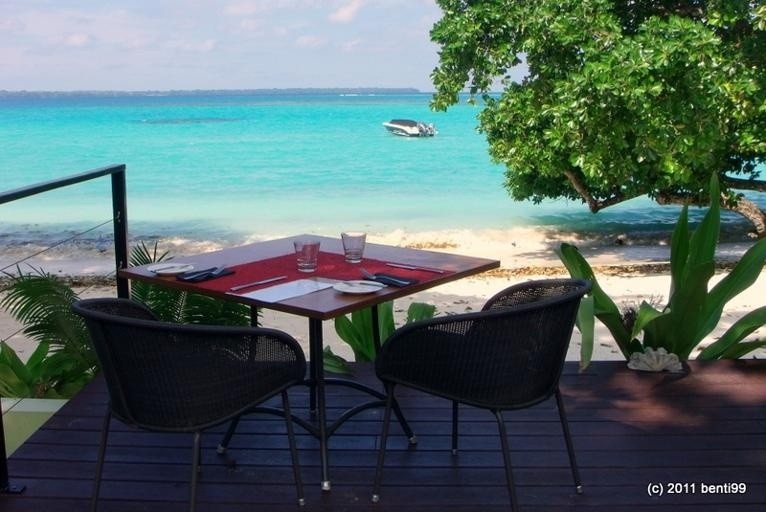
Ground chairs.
[73,296,310,512]
[372,277,592,511]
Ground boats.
[382,117,438,138]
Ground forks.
[342,281,388,288]
[183,266,225,280]
[359,267,410,285]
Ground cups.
[292,239,320,273]
[340,232,366,264]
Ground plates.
[147,264,194,276]
[332,281,385,294]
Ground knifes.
[151,265,197,272]
[229,276,287,291]
[386,264,444,275]
